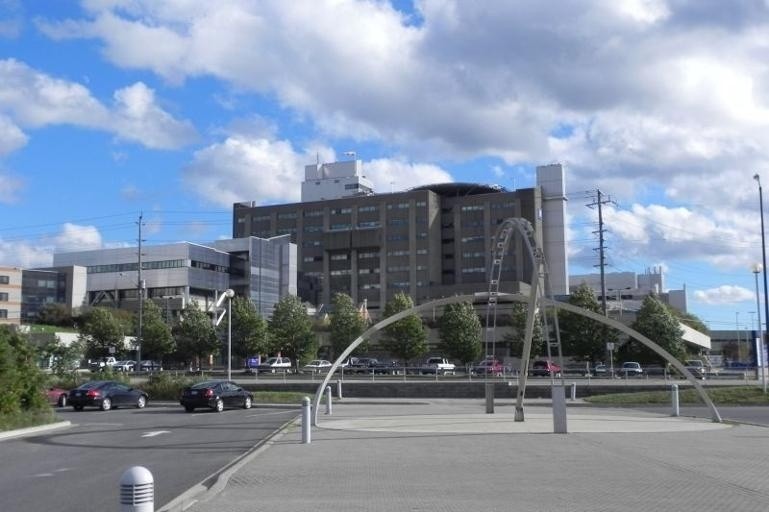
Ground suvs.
[532,359,561,376]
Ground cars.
[335,357,397,374]
[421,356,456,376]
[68,380,150,412]
[302,359,333,375]
[89,354,162,374]
[44,387,71,407]
[592,362,644,379]
[180,380,255,411]
[475,359,504,375]
[680,358,707,379]
[256,355,292,374]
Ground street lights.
[119,272,144,371]
[735,173,769,396]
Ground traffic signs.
[225,286,235,383]
